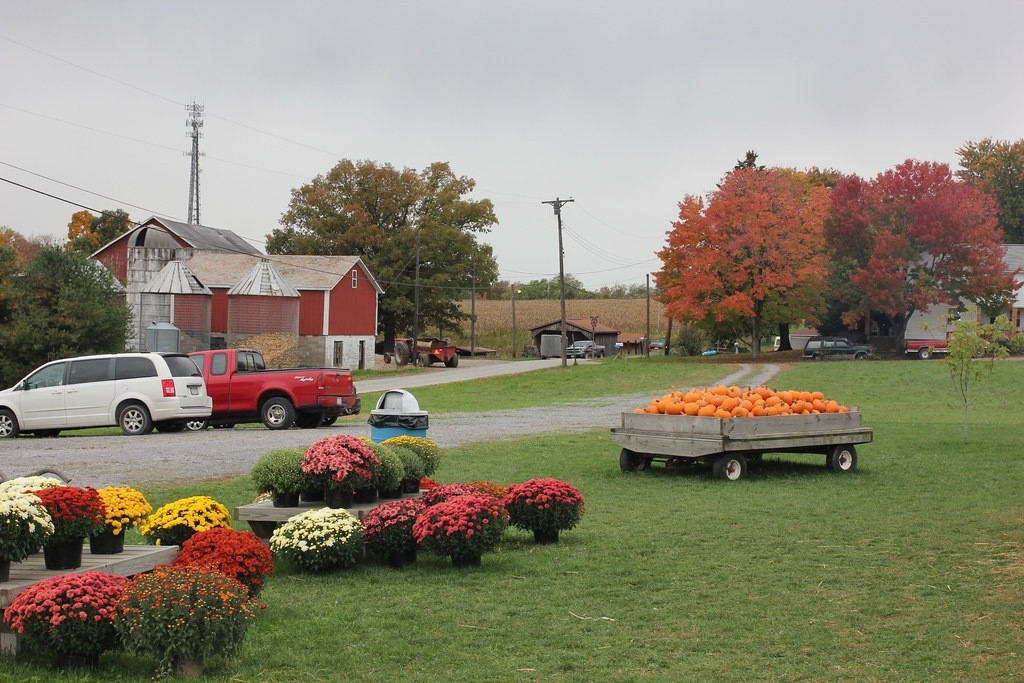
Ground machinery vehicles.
[384,336,458,368]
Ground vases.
[355,484,379,502]
[42,537,83,569]
[381,480,402,499]
[90,527,125,555]
[271,487,299,507]
[387,548,417,568]
[451,547,481,570]
[172,652,204,678]
[533,529,558,545]
[403,476,419,492]
[328,491,353,509]
[0,556,10,583]
[54,648,100,672]
[300,488,324,501]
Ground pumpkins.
[633,384,848,418]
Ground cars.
[701,346,717,356]
[650,338,667,350]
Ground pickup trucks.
[566,340,606,360]
[179,348,362,431]
[904,331,987,360]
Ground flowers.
[0,433,587,681]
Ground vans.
[773,336,781,352]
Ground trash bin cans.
[370,389,429,443]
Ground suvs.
[0,351,214,439]
[800,334,873,362]
